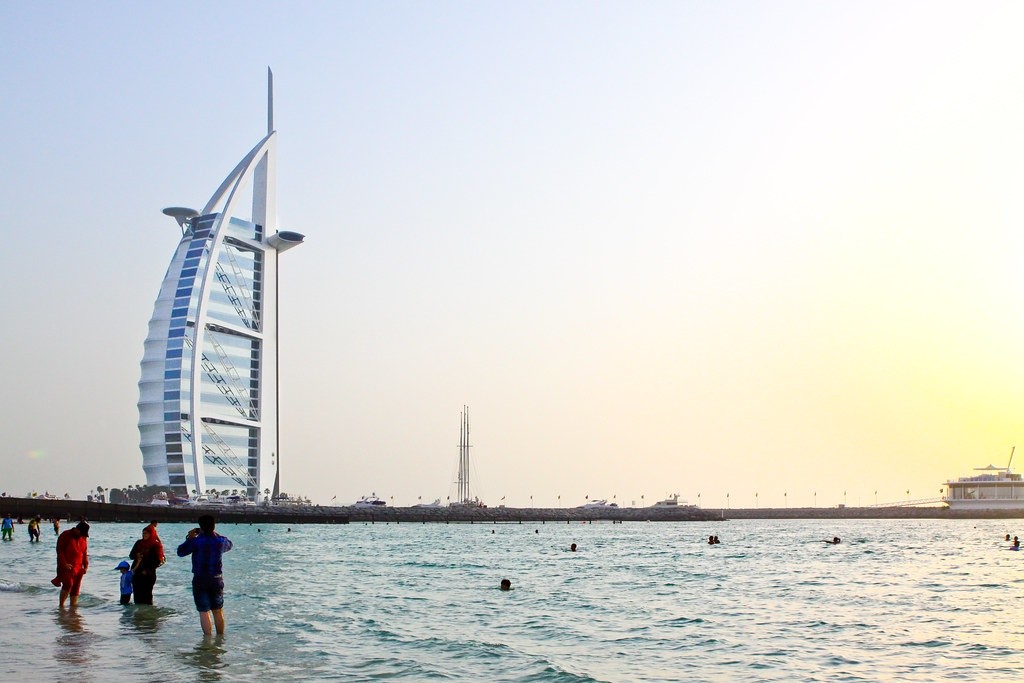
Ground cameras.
[194,528,200,534]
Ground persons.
[28,517,41,542]
[1,513,14,540]
[571,544,576,550]
[114,561,133,604]
[130,520,165,605]
[56,521,90,606]
[501,580,510,590]
[708,536,720,545]
[177,514,233,634]
[49,515,60,533]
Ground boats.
[351,497,386,508]
[585,500,619,510]
[148,493,307,506]
[942,447,1023,509]
[411,499,444,508]
[648,495,697,508]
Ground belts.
[193,574,222,578]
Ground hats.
[76,520,90,537]
[115,561,130,570]
